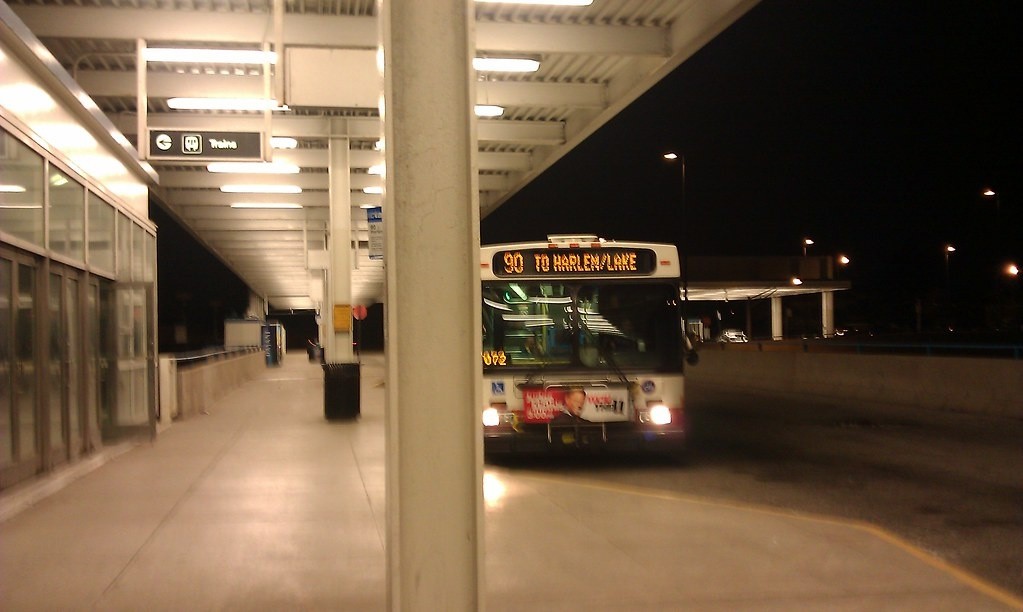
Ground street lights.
[804,235,814,280]
[661,150,687,241]
[943,242,957,339]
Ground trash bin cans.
[321,363,360,419]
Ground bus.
[479,233,699,457]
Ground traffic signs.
[146,127,264,164]
